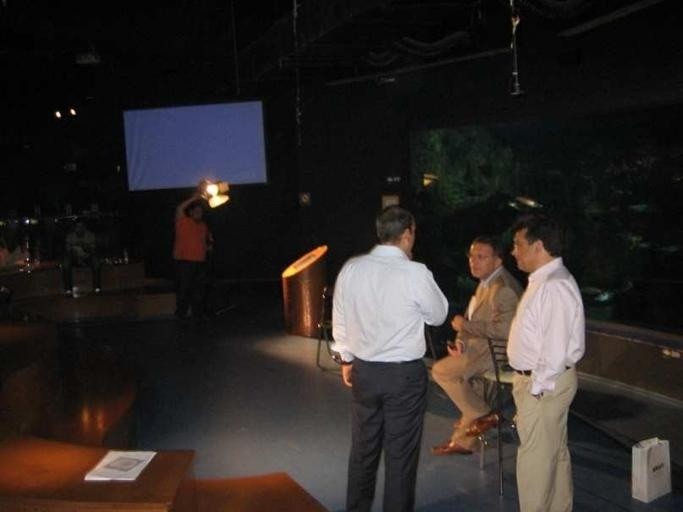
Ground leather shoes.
[432,440,472,455]
[466,410,502,437]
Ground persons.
[427,234,526,458]
[330,204,452,512]
[504,213,588,512]
[171,190,213,323]
[58,218,101,297]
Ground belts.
[517,367,570,375]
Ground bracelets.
[339,359,352,366]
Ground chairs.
[479,334,521,500]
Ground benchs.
[0,254,182,326]
[0,319,329,512]
[562,317,682,474]
